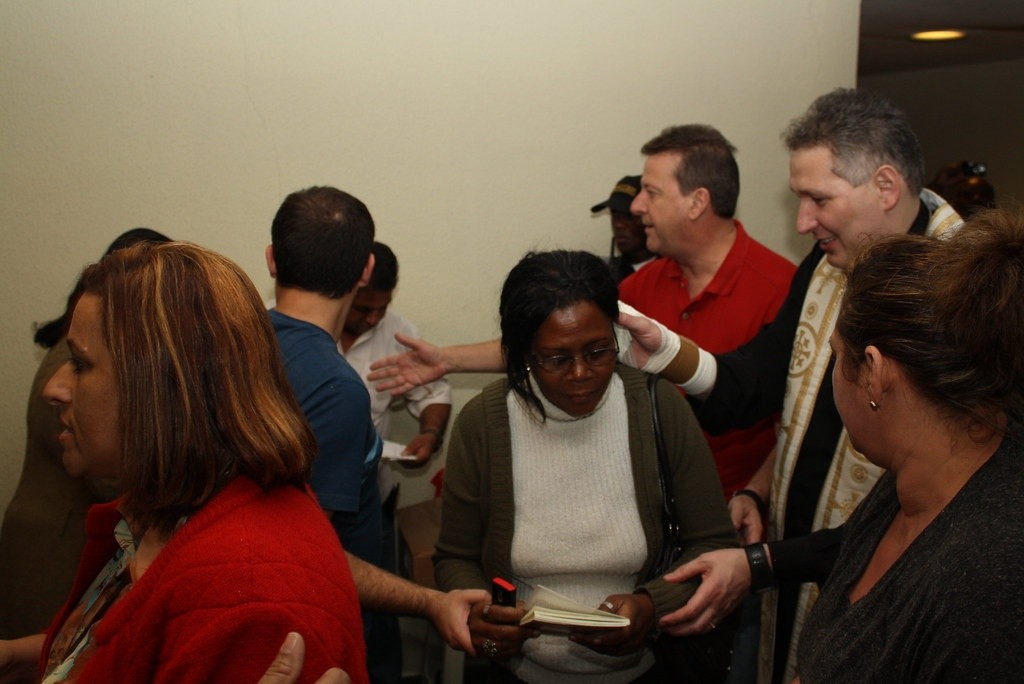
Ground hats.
[592,175,642,213]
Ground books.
[520,583,632,632]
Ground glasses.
[529,320,620,373]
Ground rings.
[480,639,497,657]
[602,601,614,613]
[710,623,716,631]
[483,605,490,619]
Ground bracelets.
[733,488,766,519]
[425,428,442,452]
[743,542,776,592]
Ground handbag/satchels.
[643,370,734,684]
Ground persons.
[0,86,1021,684]
